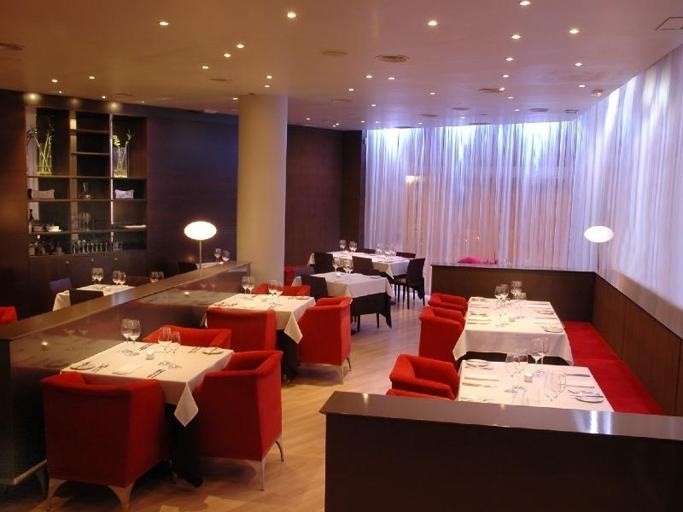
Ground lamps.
[583,215,614,272]
[180,210,220,271]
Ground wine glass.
[155,326,171,368]
[120,317,133,356]
[168,331,184,369]
[222,250,232,264]
[331,239,407,282]
[465,278,564,336]
[214,248,222,265]
[457,338,607,408]
[128,319,142,356]
[214,275,311,309]
[90,267,165,292]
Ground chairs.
[0,304,16,324]
[50,250,232,310]
[146,324,231,351]
[293,296,352,386]
[42,370,173,510]
[211,308,297,383]
[178,347,285,492]
[301,274,328,298]
[386,351,461,399]
[418,305,464,357]
[248,282,309,295]
[430,293,469,313]
[291,271,395,333]
[314,248,425,309]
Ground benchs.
[562,318,668,417]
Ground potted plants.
[112,126,134,177]
[26,112,58,175]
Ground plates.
[69,342,225,378]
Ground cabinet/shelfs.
[23,105,149,254]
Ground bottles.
[27,208,124,256]
